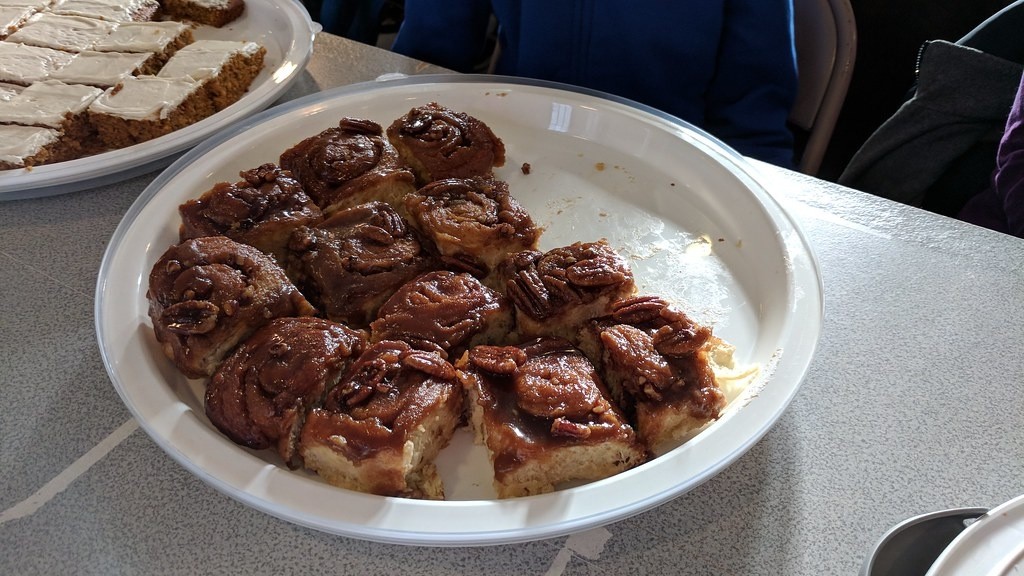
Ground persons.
[995,71,1024,241]
[390,0,798,169]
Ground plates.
[0,0,322,199]
[95,74,826,547]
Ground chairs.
[487,0,856,175]
[837,0,1024,237]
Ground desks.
[0,31,1024,576]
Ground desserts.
[150,105,734,497]
[0,0,267,169]
